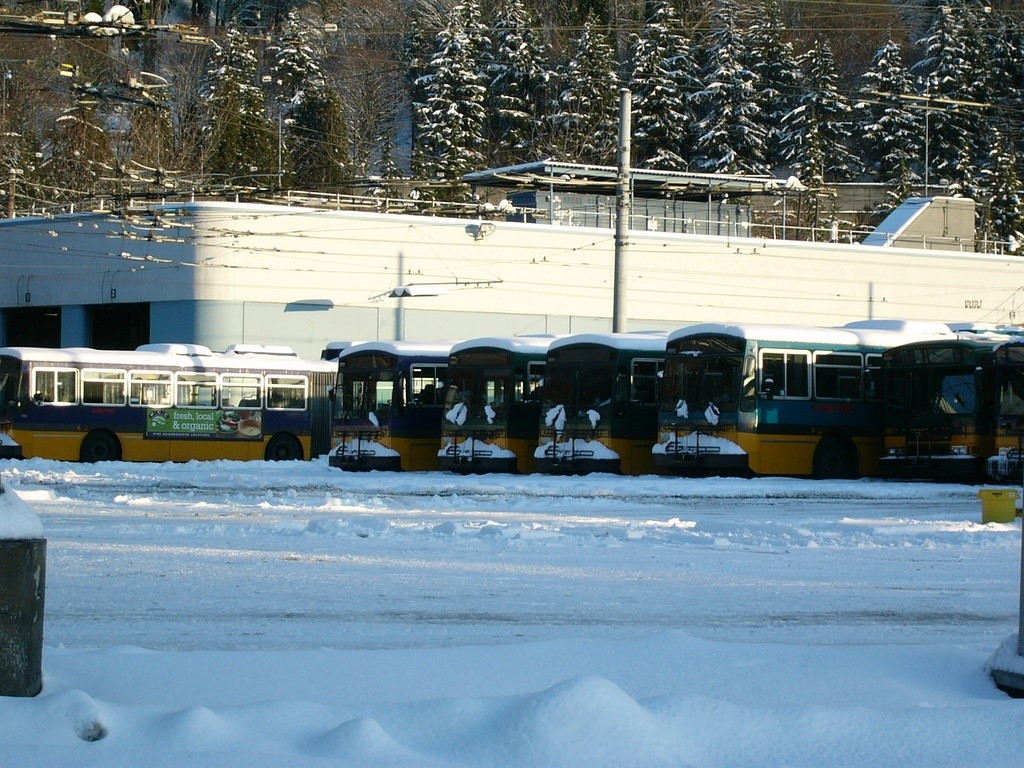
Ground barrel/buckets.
[977,489,1017,525]
[0,538,47,697]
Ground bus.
[321,328,669,478]
[652,319,1022,480]
[0,344,392,464]
[879,340,1024,485]
